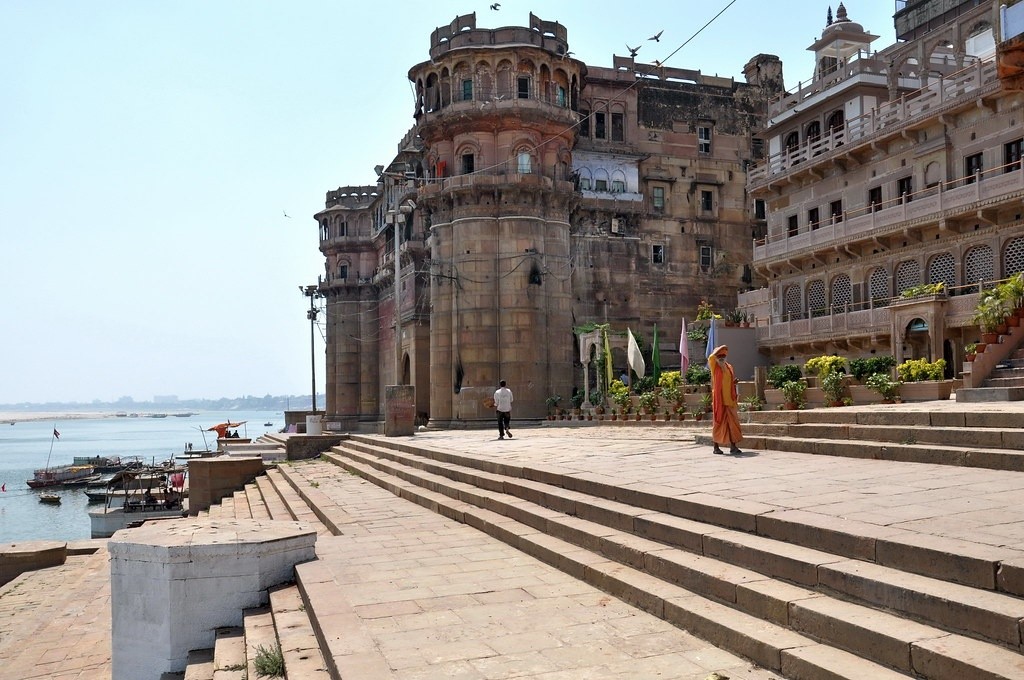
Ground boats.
[264,422,272,426]
[209,420,252,446]
[26,447,212,503]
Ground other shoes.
[730,449,742,455]
[713,449,723,454]
[498,436,504,440]
[506,431,512,438]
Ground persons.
[144,488,180,509]
[620,370,628,387]
[706,345,743,454]
[489,379,513,440]
[225,429,241,439]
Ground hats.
[713,344,728,356]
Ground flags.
[706,315,715,368]
[53,428,60,438]
[652,323,660,385]
[627,327,645,379]
[679,317,690,378]
[604,331,613,389]
